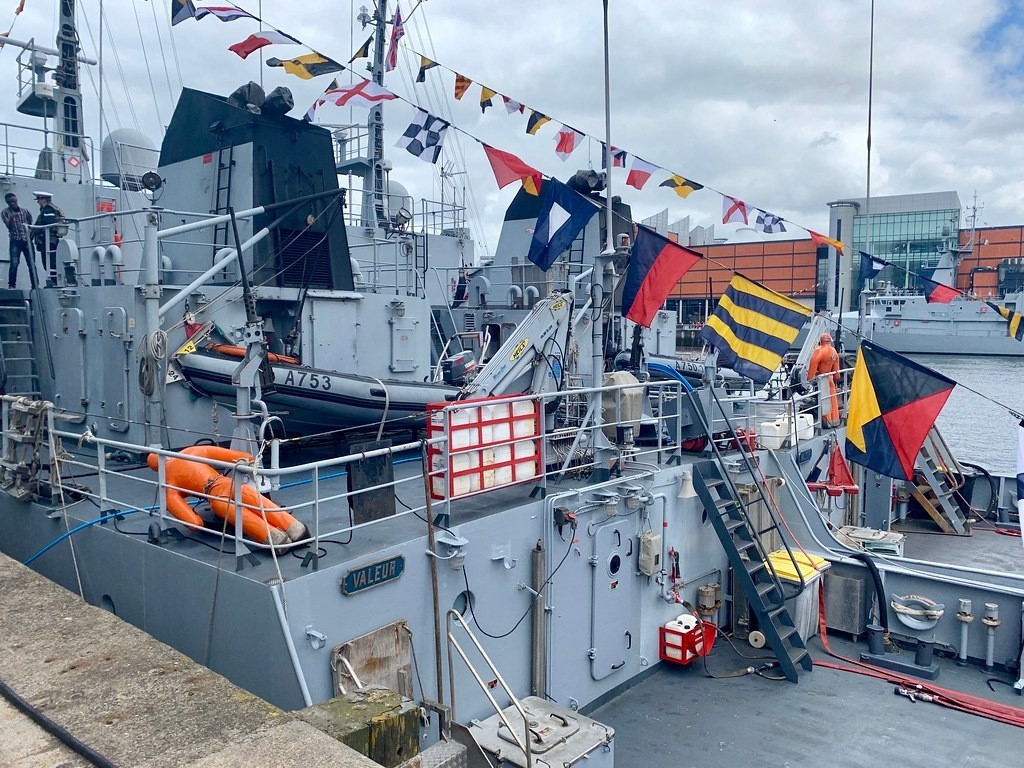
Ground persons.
[808,333,841,429]
[2,193,39,290]
[148,446,306,555]
[30,191,61,286]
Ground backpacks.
[43,202,68,237]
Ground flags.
[659,175,704,199]
[194,6,251,22]
[987,301,1024,341]
[417,57,441,83]
[722,195,753,224]
[483,145,541,196]
[228,30,301,59]
[809,230,844,255]
[395,110,449,163]
[922,277,961,303]
[526,110,551,135]
[384,5,404,71]
[455,75,472,99]
[601,142,626,169]
[846,342,953,479]
[503,96,525,114]
[553,125,585,163]
[858,251,888,278]
[324,79,397,108]
[698,273,812,385]
[529,177,602,270]
[621,225,701,327]
[480,87,496,113]
[754,210,786,233]
[267,51,345,80]
[626,158,659,190]
[172,0,196,26]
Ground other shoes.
[43,279,56,287]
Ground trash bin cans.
[757,549,833,645]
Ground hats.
[33,191,53,200]
